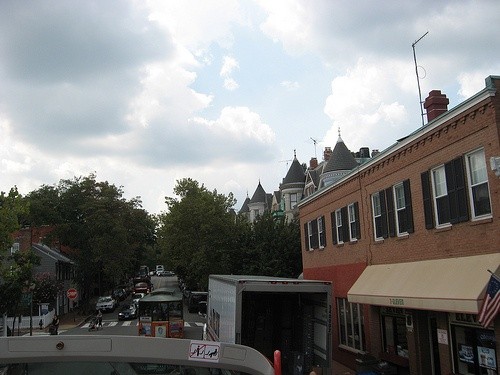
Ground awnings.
[345,252,500,316]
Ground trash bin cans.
[374,359,397,375]
[355,352,376,375]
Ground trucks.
[135,266,150,279]
[156,265,164,275]
[134,276,152,296]
[203,274,334,375]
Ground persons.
[96,309,104,329]
[52,314,61,335]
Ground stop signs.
[66,289,78,300]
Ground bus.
[137,289,184,339]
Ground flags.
[479,273,500,328]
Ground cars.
[96,295,117,312]
[109,276,136,302]
[178,273,198,297]
[149,272,154,277]
[118,300,139,321]
[161,271,175,276]
[132,293,144,300]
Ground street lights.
[28,223,50,336]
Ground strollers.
[49,323,59,336]
[88,317,99,332]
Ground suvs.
[189,292,208,314]
